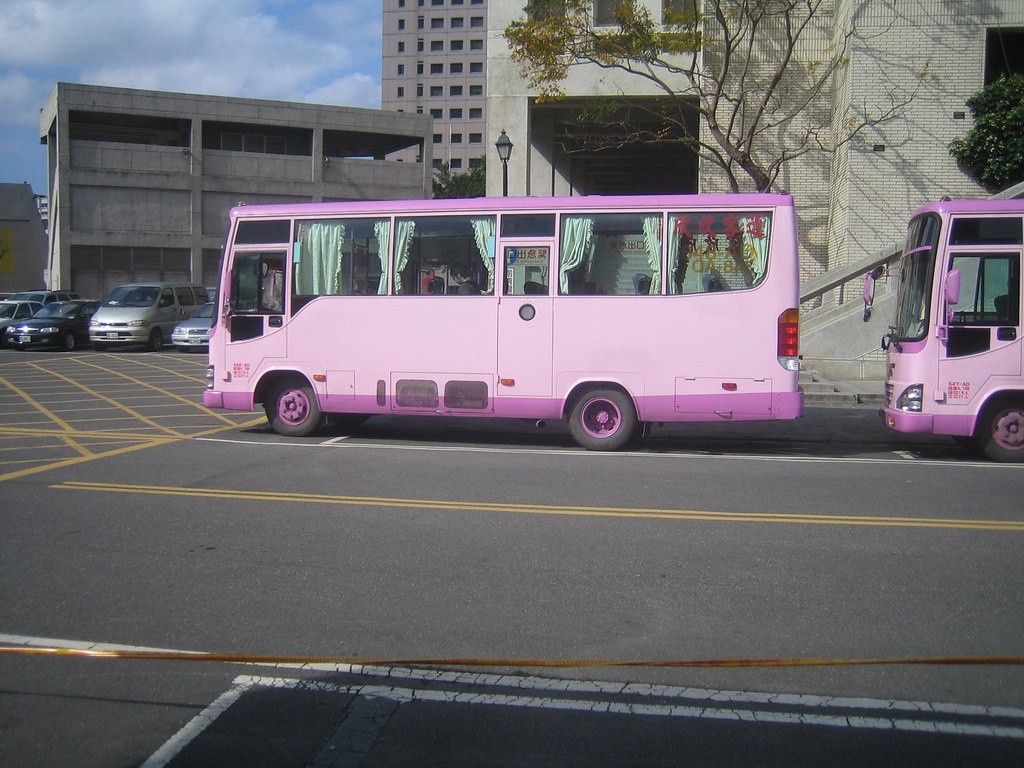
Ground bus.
[203,189,804,452]
[862,196,1024,463]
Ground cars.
[6,289,81,305]
[5,300,103,352]
[0,300,44,333]
[206,287,216,301]
[171,302,215,352]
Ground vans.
[88,282,209,351]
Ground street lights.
[495,128,514,197]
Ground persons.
[450,262,480,294]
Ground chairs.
[523,273,724,295]
